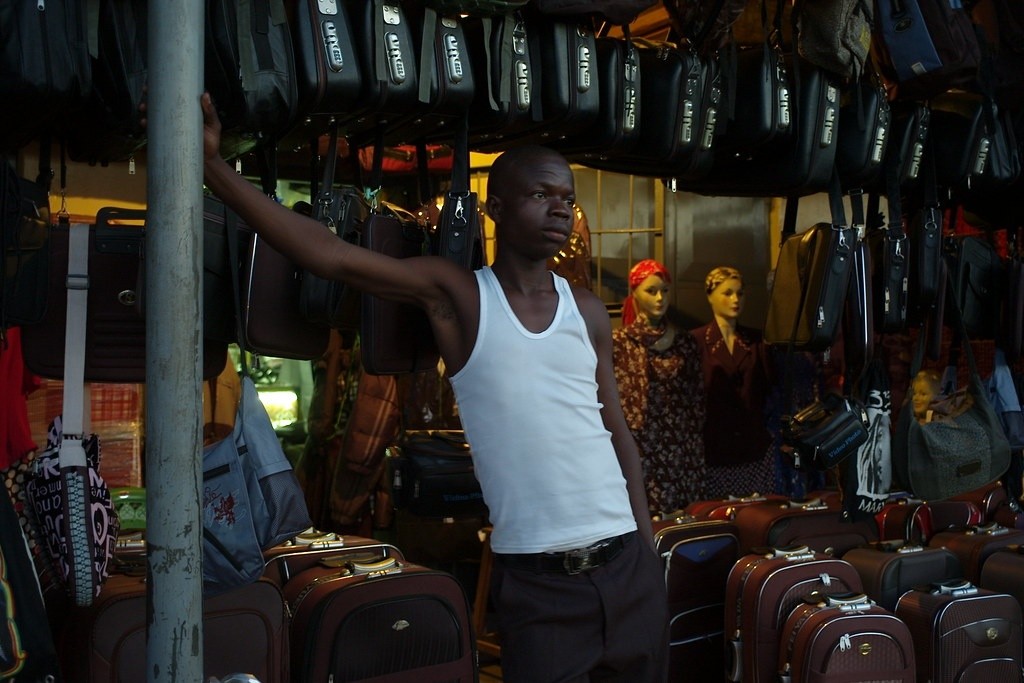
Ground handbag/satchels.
[0,0,1024,576]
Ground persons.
[613,259,781,521]
[137,92,672,683]
[912,369,956,426]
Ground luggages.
[647,495,1024,683]
[82,525,483,683]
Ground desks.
[395,511,487,568]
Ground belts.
[494,533,634,576]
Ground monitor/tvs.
[253,385,301,429]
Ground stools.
[473,527,500,659]
